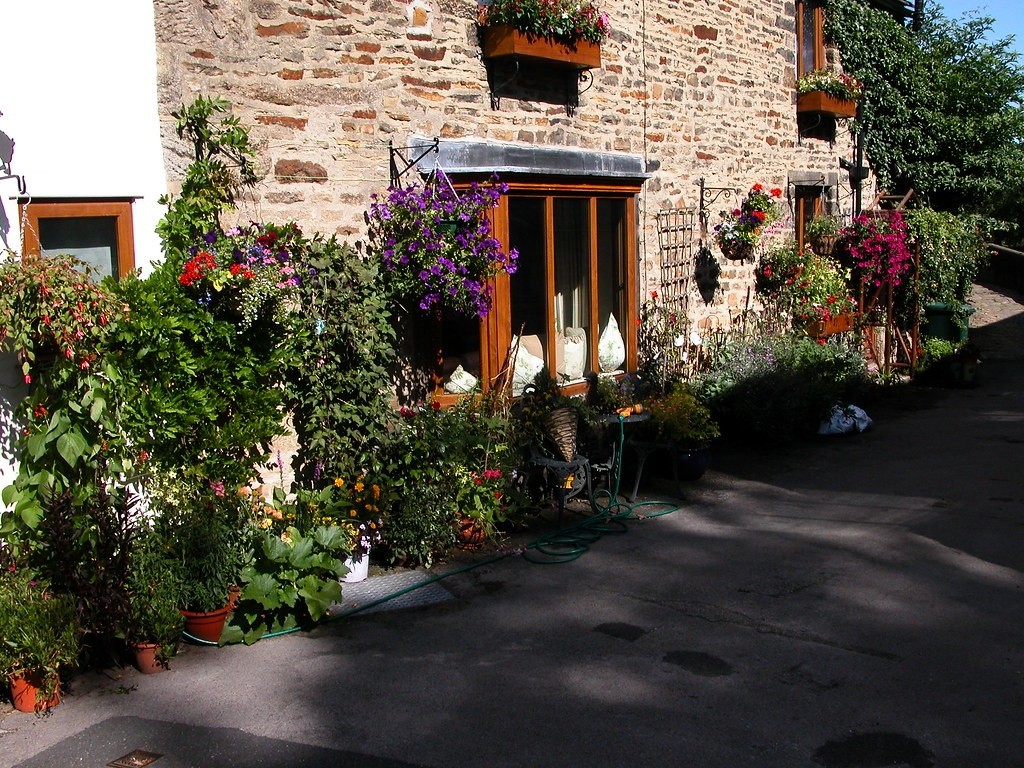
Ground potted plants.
[1,2,924,720]
